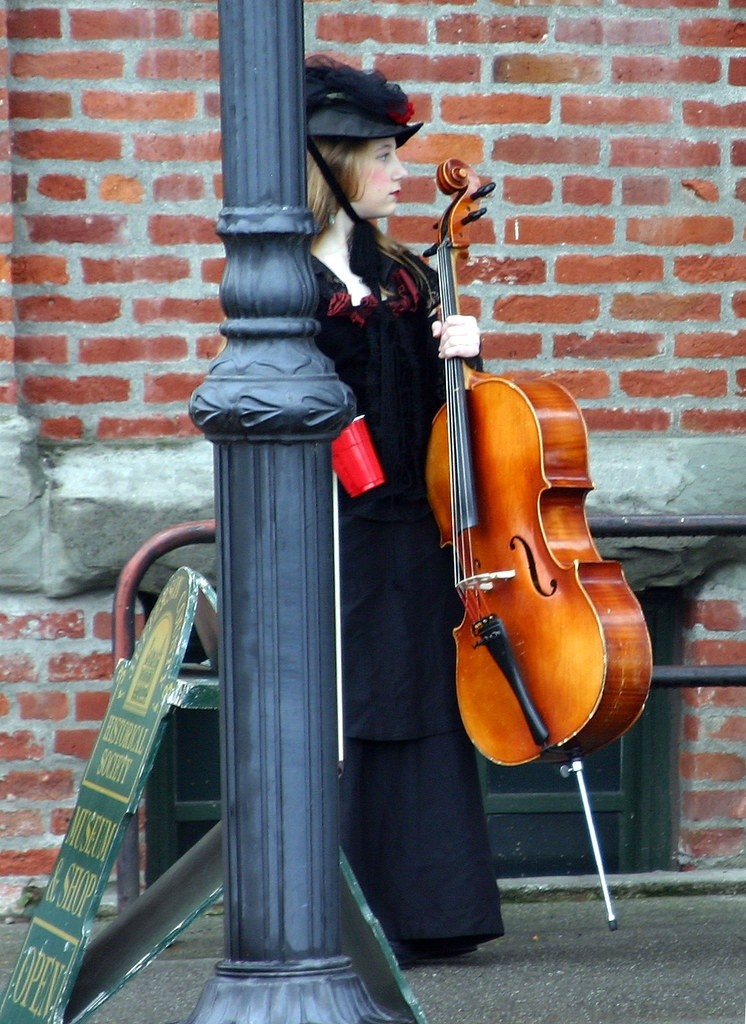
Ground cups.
[332,413,388,498]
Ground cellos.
[417,156,657,936]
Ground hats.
[301,53,425,148]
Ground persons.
[299,55,507,970]
